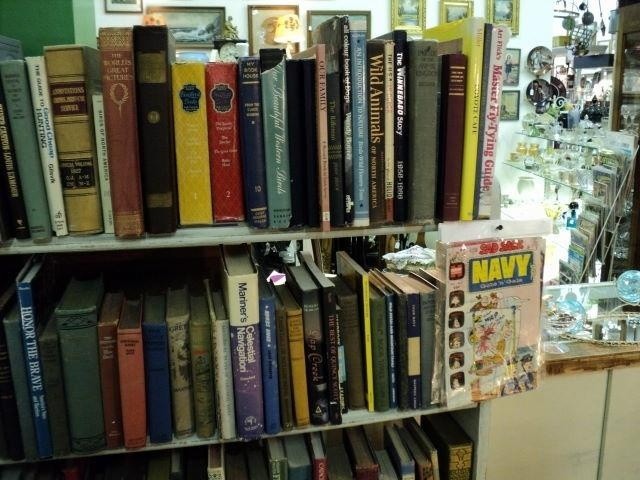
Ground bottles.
[508,75,610,228]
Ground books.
[0,13,506,244]
[1,233,472,480]
[548,131,639,287]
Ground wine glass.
[618,104,639,134]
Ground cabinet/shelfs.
[501,130,638,280]
[0,220,501,480]
[615,34,639,254]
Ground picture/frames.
[440,0,474,26]
[247,4,299,57]
[488,0,519,35]
[391,0,425,34]
[273,14,302,56]
[500,90,520,121]
[307,10,371,51]
[504,49,521,87]
[147,6,226,42]
[105,1,142,13]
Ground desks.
[484,281,640,479]
